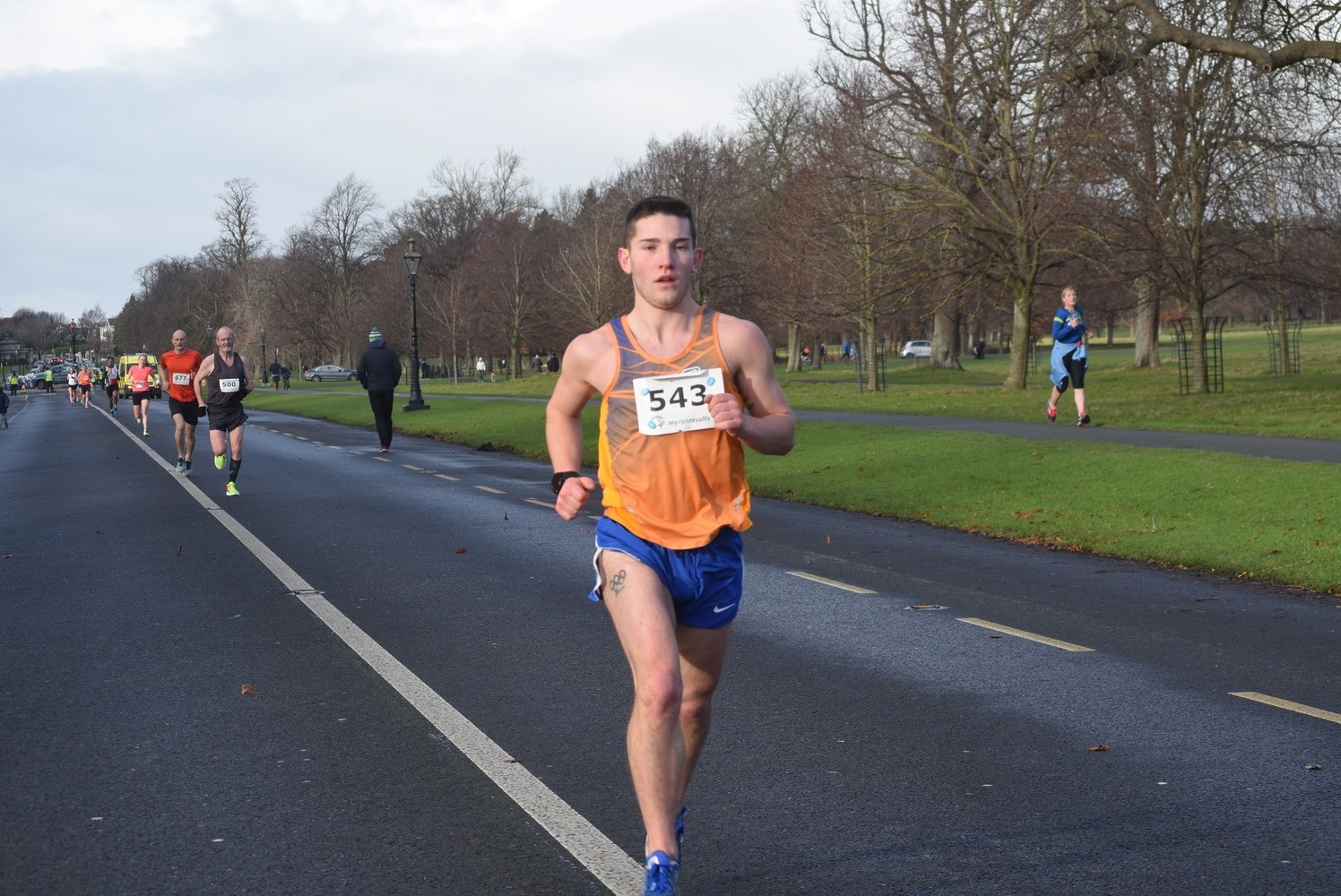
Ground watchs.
[551,471,580,495]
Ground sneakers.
[1076,412,1090,428]
[644,850,680,896]
[645,807,688,870]
[175,458,185,473]
[1047,399,1057,422]
[183,460,192,477]
[226,482,240,496]
[214,440,228,470]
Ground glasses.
[217,336,235,342]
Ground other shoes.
[114,407,118,414]
[137,418,142,423]
[143,431,149,436]
[111,411,114,416]
[379,445,388,452]
[69,398,89,410]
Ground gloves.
[197,407,206,417]
[238,389,247,400]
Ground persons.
[9,371,19,396]
[126,355,157,436]
[159,329,204,478]
[803,340,856,363]
[269,359,291,390]
[545,196,798,895]
[67,364,107,409]
[530,353,559,375]
[44,367,55,394]
[422,359,431,380]
[103,356,120,416]
[1047,287,1094,428]
[194,327,255,496]
[477,357,486,383]
[357,326,402,452]
[975,334,986,359]
[0,385,10,429]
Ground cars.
[898,339,933,359]
[303,364,359,382]
[4,351,163,400]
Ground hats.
[369,327,382,342]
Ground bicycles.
[845,353,856,365]
[820,352,834,364]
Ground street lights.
[206,321,214,355]
[258,328,269,383]
[401,235,431,412]
[57,318,89,364]
